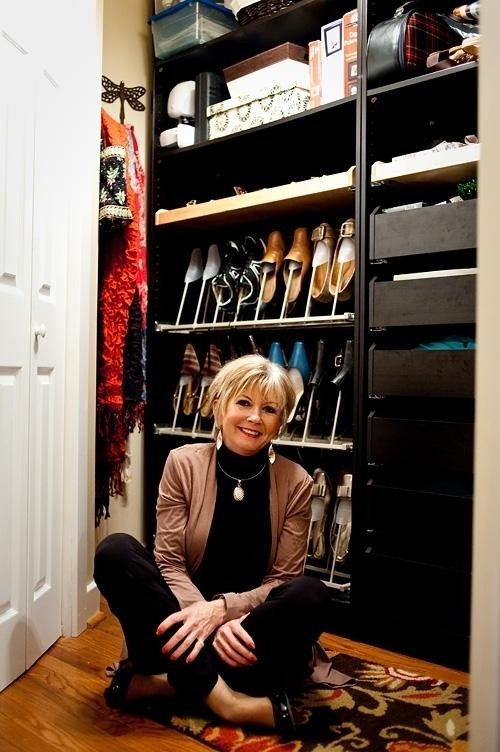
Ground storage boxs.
[148,1,238,58]
[206,81,310,138]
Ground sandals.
[304,468,334,561]
[308,338,329,430]
[209,215,357,313]
[172,341,304,427]
[103,664,142,711]
[264,690,298,734]
[329,474,359,562]
[329,339,356,429]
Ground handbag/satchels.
[364,1,463,87]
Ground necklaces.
[216,459,271,503]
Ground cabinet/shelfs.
[363,1,481,668]
[145,0,362,623]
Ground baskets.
[237,1,300,26]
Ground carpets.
[103,647,469,751]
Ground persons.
[93,353,332,733]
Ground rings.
[196,637,205,648]
